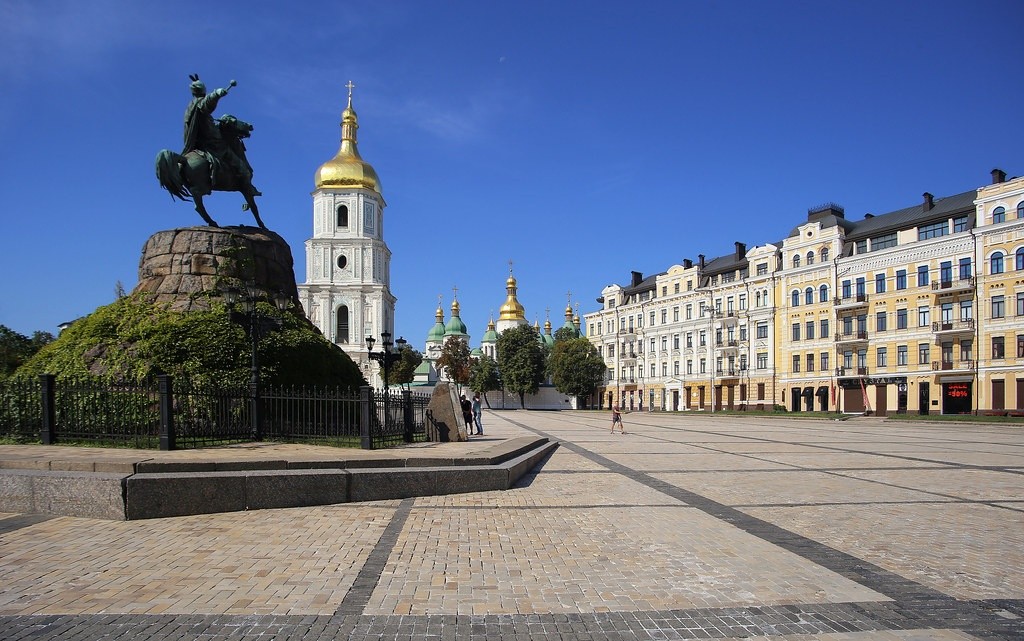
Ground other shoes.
[474,432,483,435]
[622,431,624,434]
[470,432,473,435]
[610,431,613,434]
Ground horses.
[156,114,267,229]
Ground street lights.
[222,277,291,445]
[702,305,717,415]
[365,328,407,433]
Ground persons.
[461,395,473,435]
[183,73,262,196]
[472,392,483,435]
[610,401,624,434]
